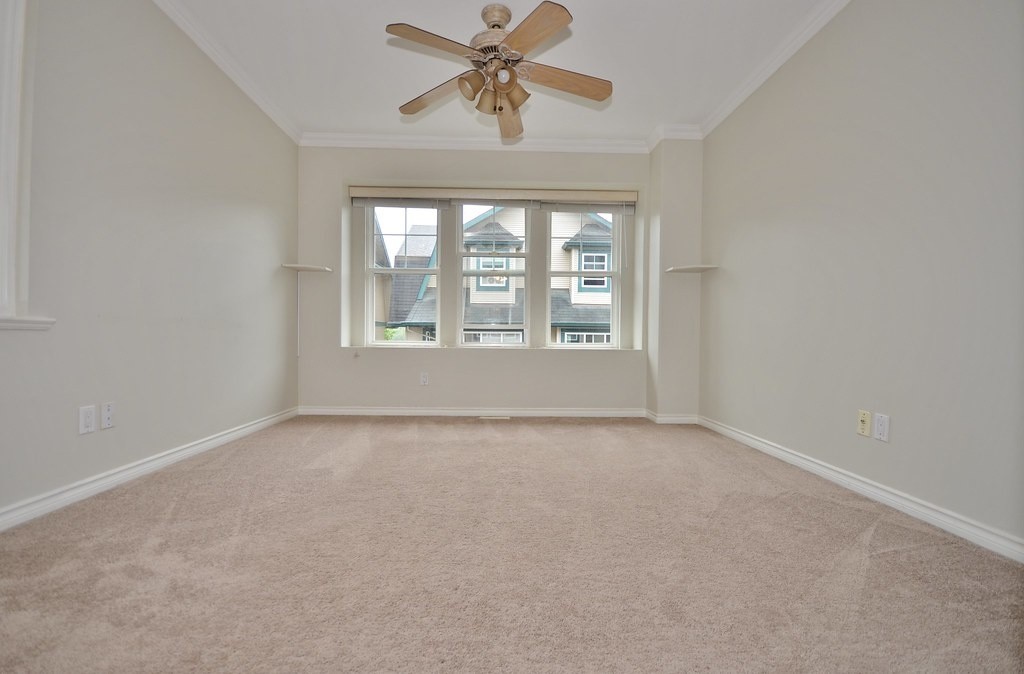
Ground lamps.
[459,59,531,116]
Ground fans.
[385,0,613,138]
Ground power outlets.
[874,413,890,442]
[78,405,96,435]
[101,401,116,429]
[857,409,872,438]
